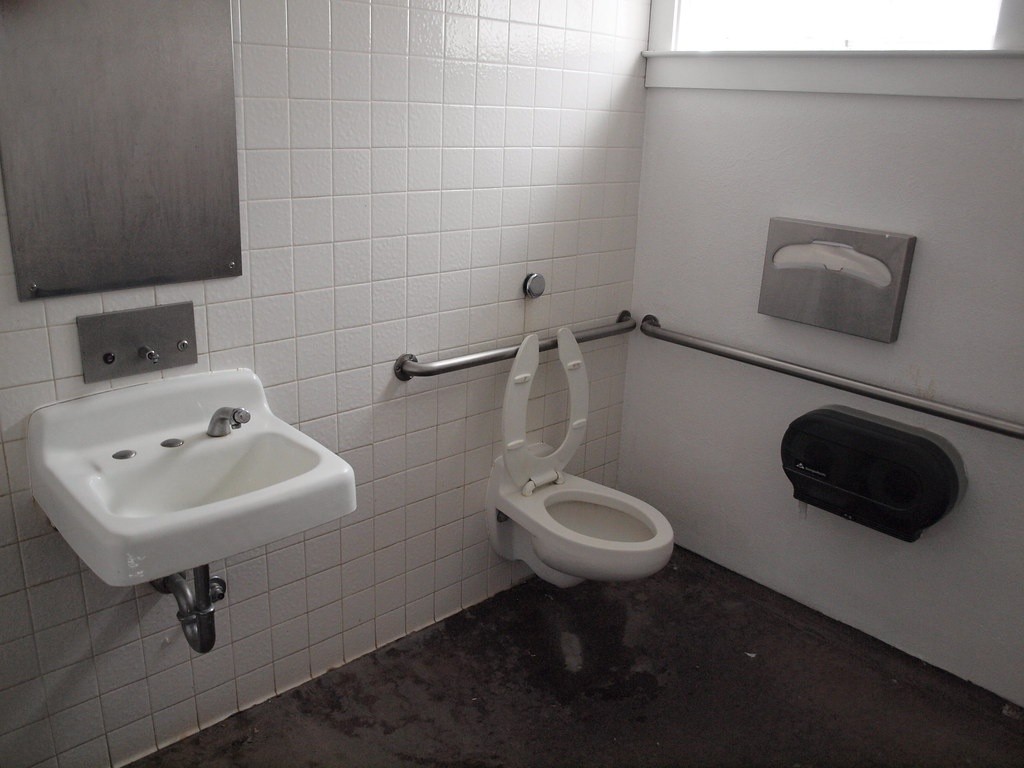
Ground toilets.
[482,326,676,590]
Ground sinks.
[22,367,359,589]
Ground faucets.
[206,406,252,438]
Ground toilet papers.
[780,403,968,544]
